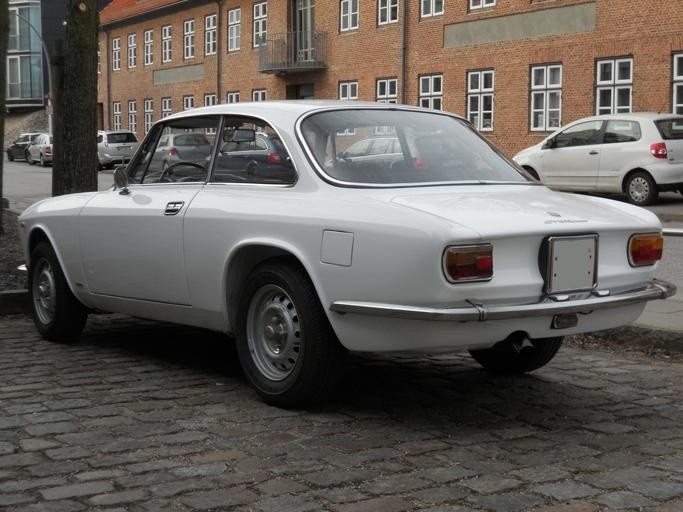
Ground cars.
[6,134,38,161]
[15,98,678,407]
[96,129,139,171]
[511,102,682,205]
[25,134,54,166]
